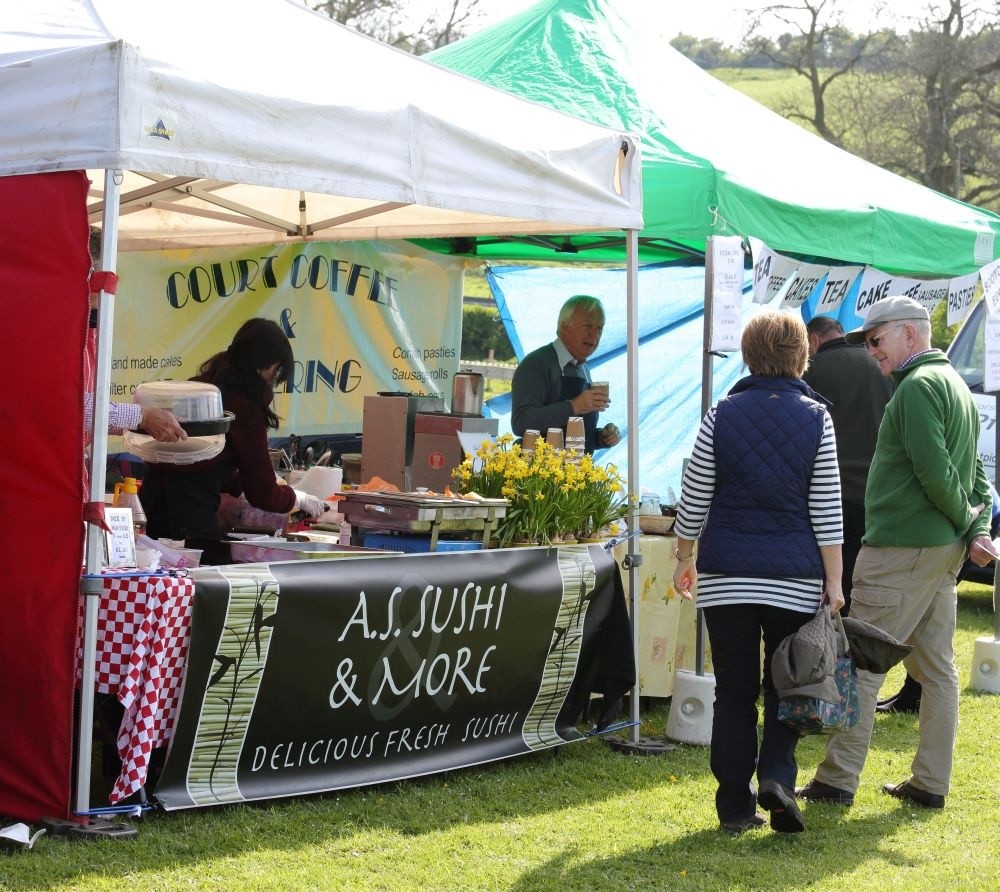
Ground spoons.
[304,446,314,470]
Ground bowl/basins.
[624,513,675,534]
[163,546,205,571]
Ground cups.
[521,429,541,463]
[591,381,609,409]
[546,427,564,471]
[565,416,586,472]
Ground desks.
[97,540,620,813]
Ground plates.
[376,391,412,397]
[122,410,236,465]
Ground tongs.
[315,450,332,466]
[279,447,294,470]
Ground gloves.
[292,489,324,519]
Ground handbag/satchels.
[777,604,859,735]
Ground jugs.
[450,367,485,417]
[639,493,663,516]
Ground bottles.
[113,476,147,540]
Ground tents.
[409,0,1000,694]
[0,0,643,833]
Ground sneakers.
[882,780,944,809]
[793,778,854,806]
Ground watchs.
[675,548,695,561]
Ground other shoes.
[875,693,921,714]
[719,809,768,833]
[758,778,804,833]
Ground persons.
[670,294,1000,835]
[83,392,188,444]
[135,318,324,566]
[510,295,622,459]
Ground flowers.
[449,433,638,537]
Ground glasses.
[863,323,908,350]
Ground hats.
[844,295,929,345]
